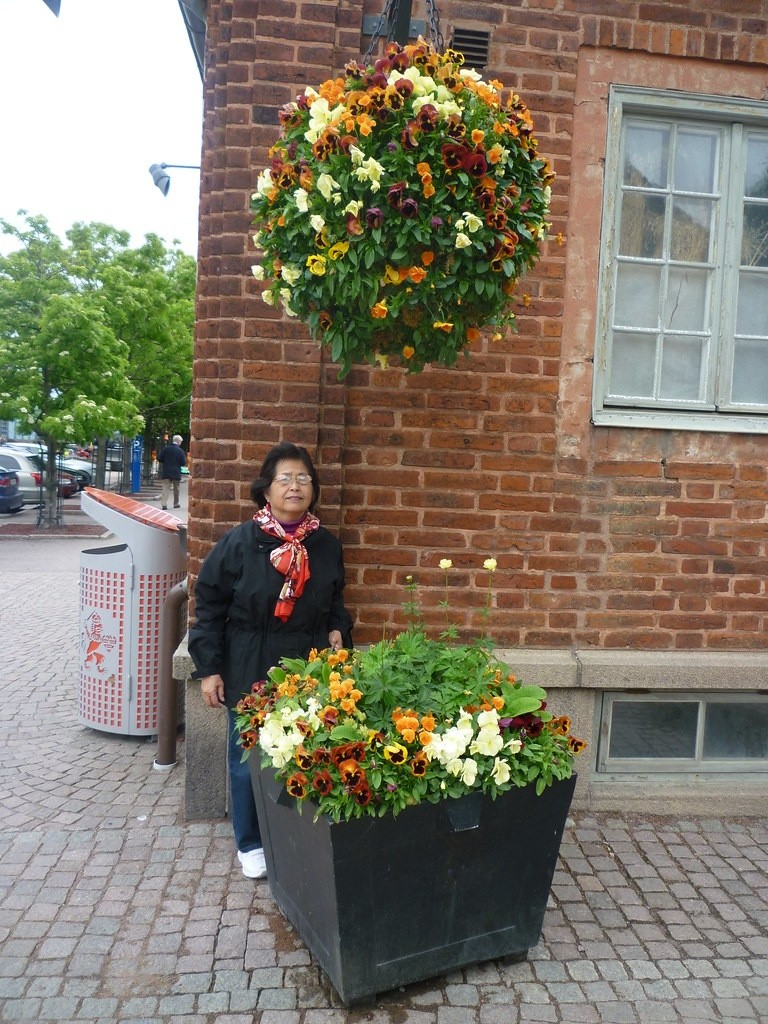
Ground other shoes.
[174,505,180,508]
[162,506,168,510]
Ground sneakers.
[238,848,268,878]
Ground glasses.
[273,475,312,484]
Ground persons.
[188,445,353,879]
[156,435,186,510]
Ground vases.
[246,744,576,1004]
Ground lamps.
[149,163,200,196]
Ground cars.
[0,446,59,505]
[54,465,92,492]
[55,469,78,499]
[5,442,97,486]
[0,466,24,512]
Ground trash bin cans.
[76,485,188,736]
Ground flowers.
[249,34,554,383]
[229,558,586,826]
